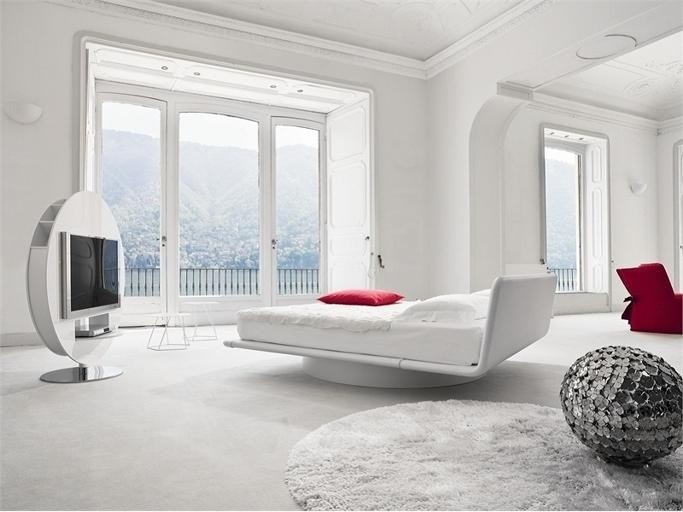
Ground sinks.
[616,264,682,334]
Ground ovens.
[223,274,557,390]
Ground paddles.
[283,399,682,511]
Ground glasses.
[3,100,43,123]
[630,182,647,194]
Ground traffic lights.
[390,293,488,327]
[318,289,405,305]
[473,289,490,297]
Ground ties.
[59,232,121,321]
